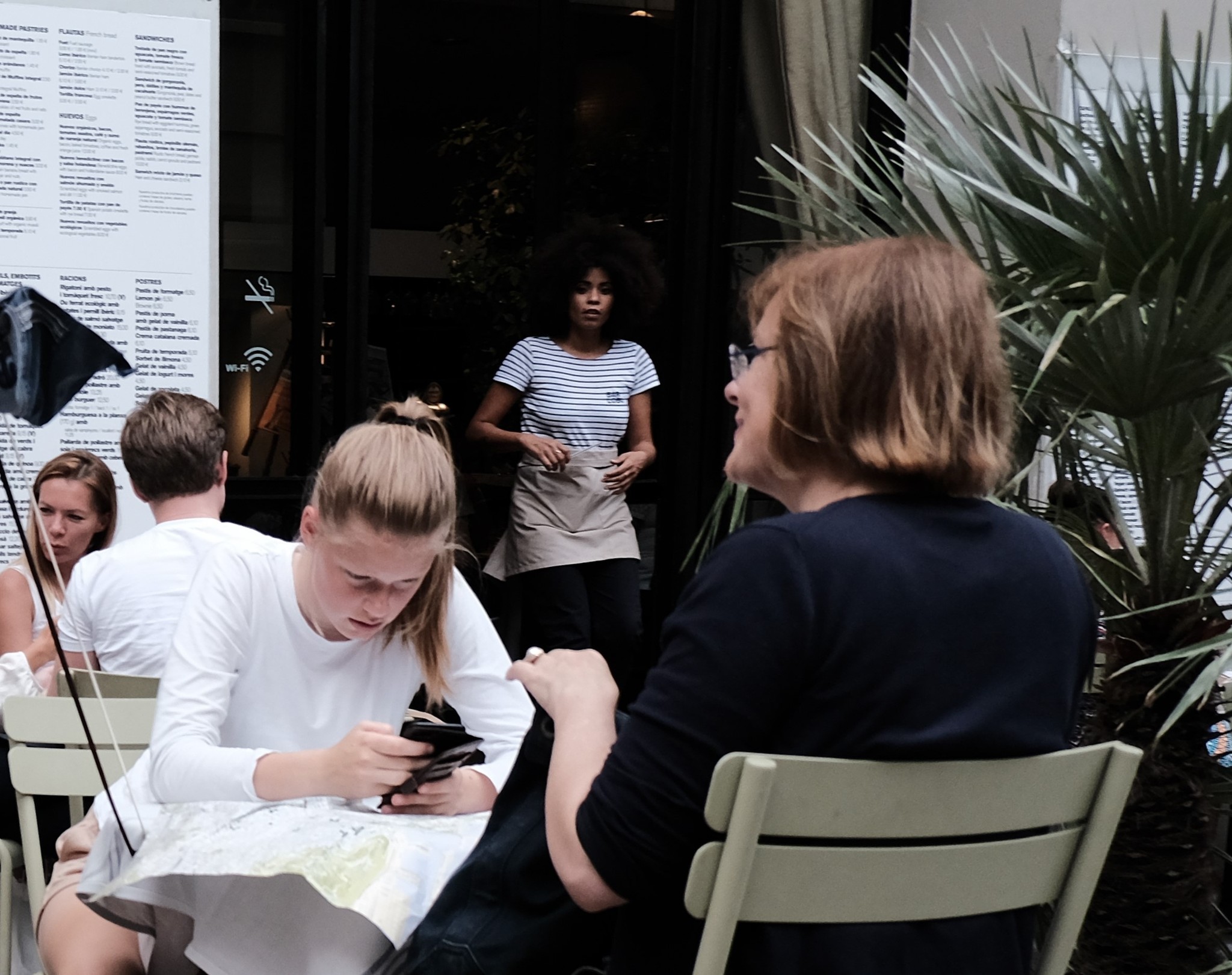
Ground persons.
[34,392,532,975]
[1042,474,1232,768]
[57,390,303,717]
[397,220,1109,975]
[469,254,657,578]
[0,448,131,898]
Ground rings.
[554,450,562,454]
[532,650,545,663]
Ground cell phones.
[378,721,466,810]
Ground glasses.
[729,342,780,384]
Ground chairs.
[685,741,1143,975]
[0,667,160,975]
